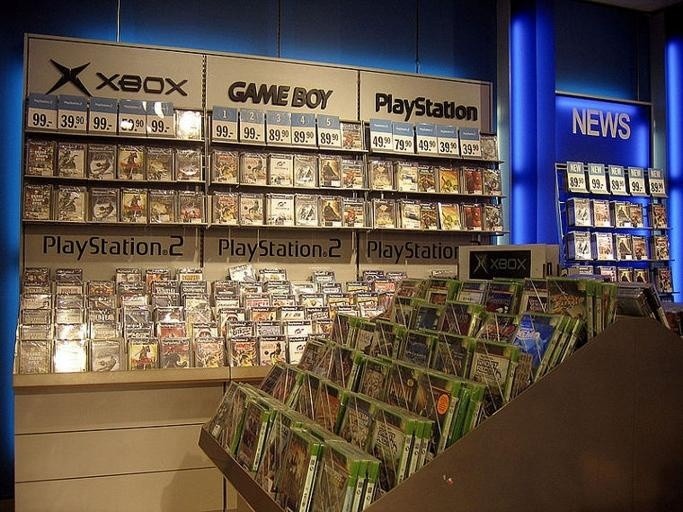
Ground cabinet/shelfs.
[554,160,681,303]
[198,281,682,511]
[12,34,511,511]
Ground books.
[16,267,224,375]
[604,280,682,336]
[224,262,362,368]
[363,271,409,319]
[206,276,622,511]
[366,157,504,231]
[21,136,204,225]
[564,160,674,303]
[209,148,365,229]
